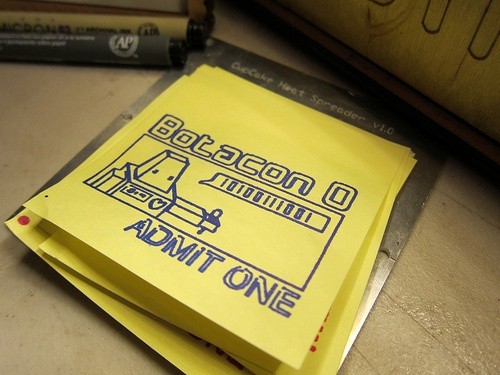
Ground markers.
[0,8,207,49]
[0,30,189,70]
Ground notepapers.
[4,64,417,375]
